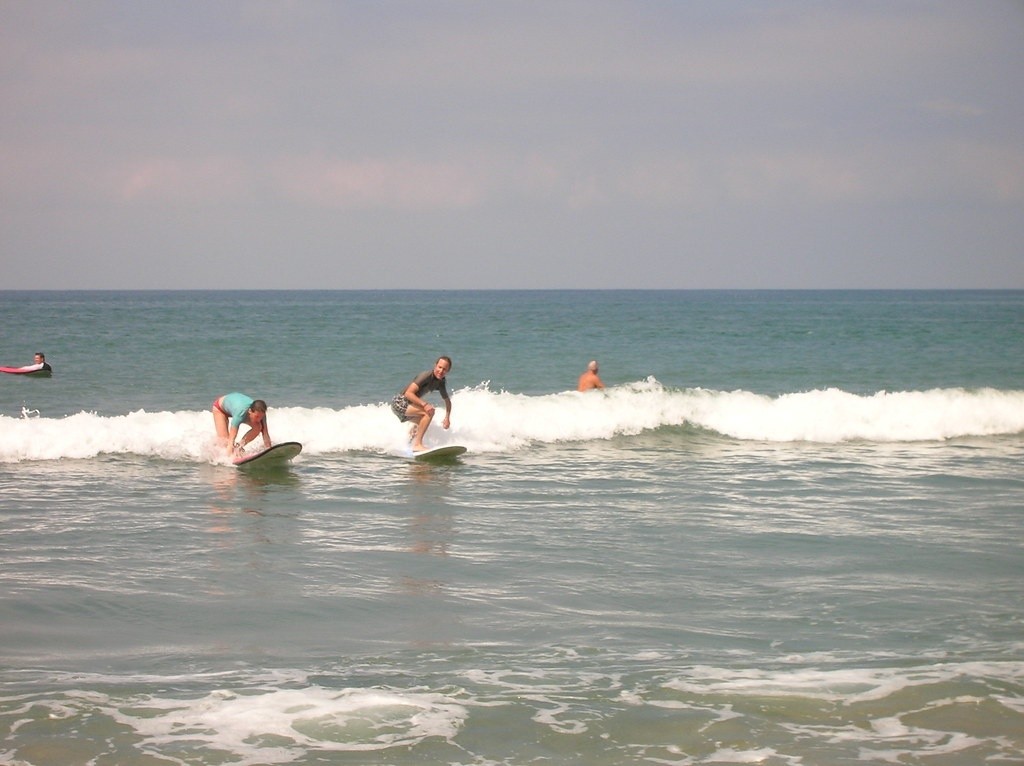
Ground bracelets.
[422,403,428,408]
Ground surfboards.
[408,444,468,462]
[231,441,303,467]
[0,365,53,379]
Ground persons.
[211,391,272,458]
[576,360,607,391]
[390,355,452,453]
[21,352,51,371]
